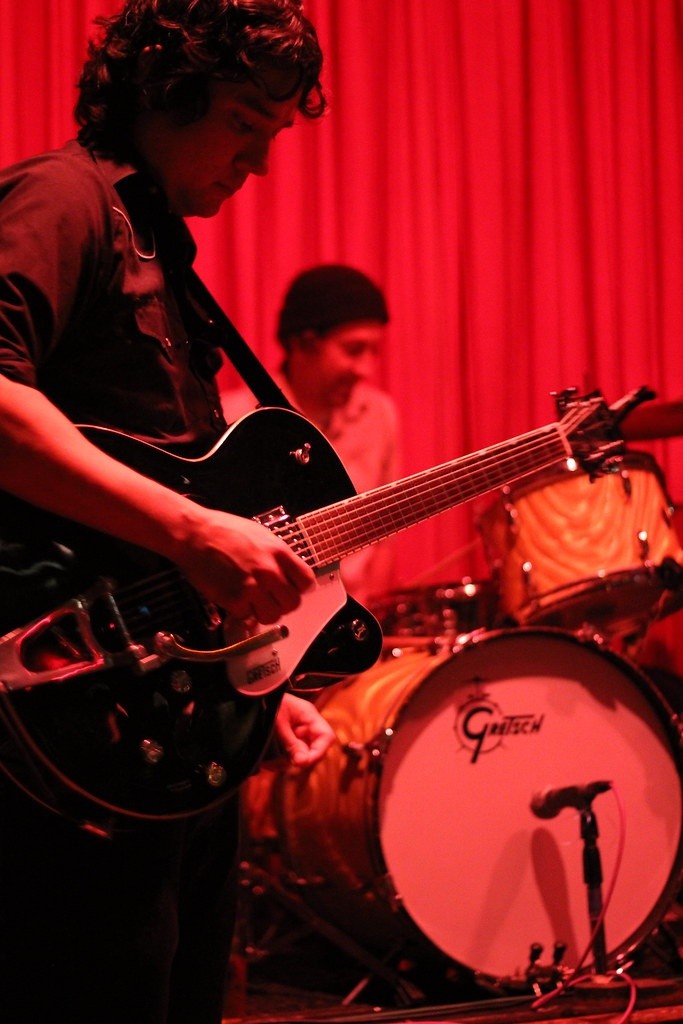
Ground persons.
[222,264,402,601]
[0,0,335,1024]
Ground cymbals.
[618,397,683,441]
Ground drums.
[270,622,683,987]
[475,447,683,640]
[376,579,489,663]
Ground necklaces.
[111,199,158,259]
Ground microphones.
[530,780,615,819]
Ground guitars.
[1,381,658,845]
